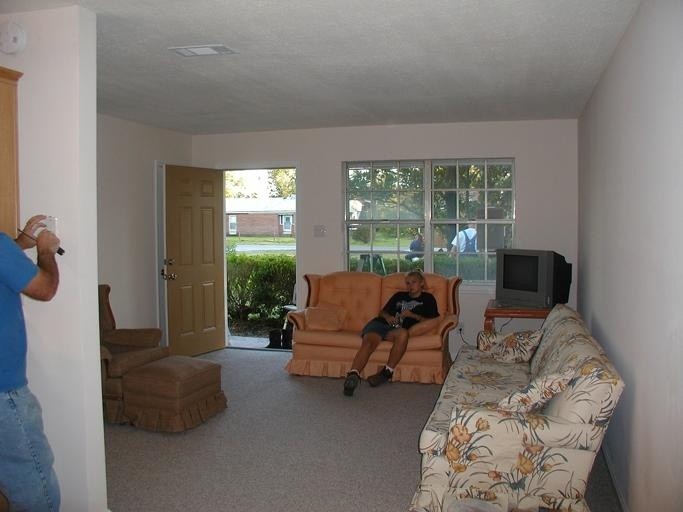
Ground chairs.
[99,285,166,430]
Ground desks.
[484,299,552,333]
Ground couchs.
[409,297,627,511]
[284,272,461,386]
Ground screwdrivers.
[17,228,65,255]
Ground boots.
[266,329,282,348]
[281,334,293,350]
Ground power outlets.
[454,320,463,335]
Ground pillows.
[313,301,347,331]
[490,328,544,365]
[492,367,573,414]
[402,312,443,335]
[305,306,333,332]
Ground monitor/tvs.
[494,247,572,309]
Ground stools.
[117,353,226,434]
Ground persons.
[1,214,62,512]
[403,233,424,259]
[448,219,478,258]
[344,270,442,394]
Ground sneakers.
[367,366,393,387]
[343,371,361,397]
